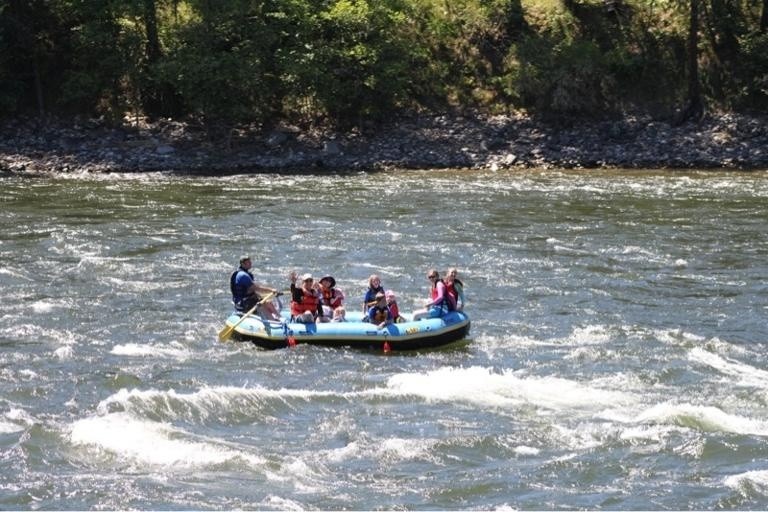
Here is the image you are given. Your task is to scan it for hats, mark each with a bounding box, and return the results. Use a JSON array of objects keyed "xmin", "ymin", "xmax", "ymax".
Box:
[
  {"xmin": 320, "ymin": 275, "xmax": 335, "ymax": 288},
  {"xmin": 303, "ymin": 274, "xmax": 313, "ymax": 281}
]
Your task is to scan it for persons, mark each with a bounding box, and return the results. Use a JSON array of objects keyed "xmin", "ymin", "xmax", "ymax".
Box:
[{"xmin": 230, "ymin": 254, "xmax": 467, "ymax": 330}]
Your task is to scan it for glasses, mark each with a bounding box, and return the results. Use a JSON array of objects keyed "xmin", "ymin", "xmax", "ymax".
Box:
[{"xmin": 429, "ymin": 276, "xmax": 435, "ymax": 278}]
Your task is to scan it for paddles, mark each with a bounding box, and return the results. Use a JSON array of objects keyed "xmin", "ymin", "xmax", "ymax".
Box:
[{"xmin": 219, "ymin": 291, "xmax": 272, "ymax": 342}]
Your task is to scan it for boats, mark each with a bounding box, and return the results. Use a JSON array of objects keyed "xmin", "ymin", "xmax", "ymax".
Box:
[{"xmin": 225, "ymin": 306, "xmax": 474, "ymax": 352}]
